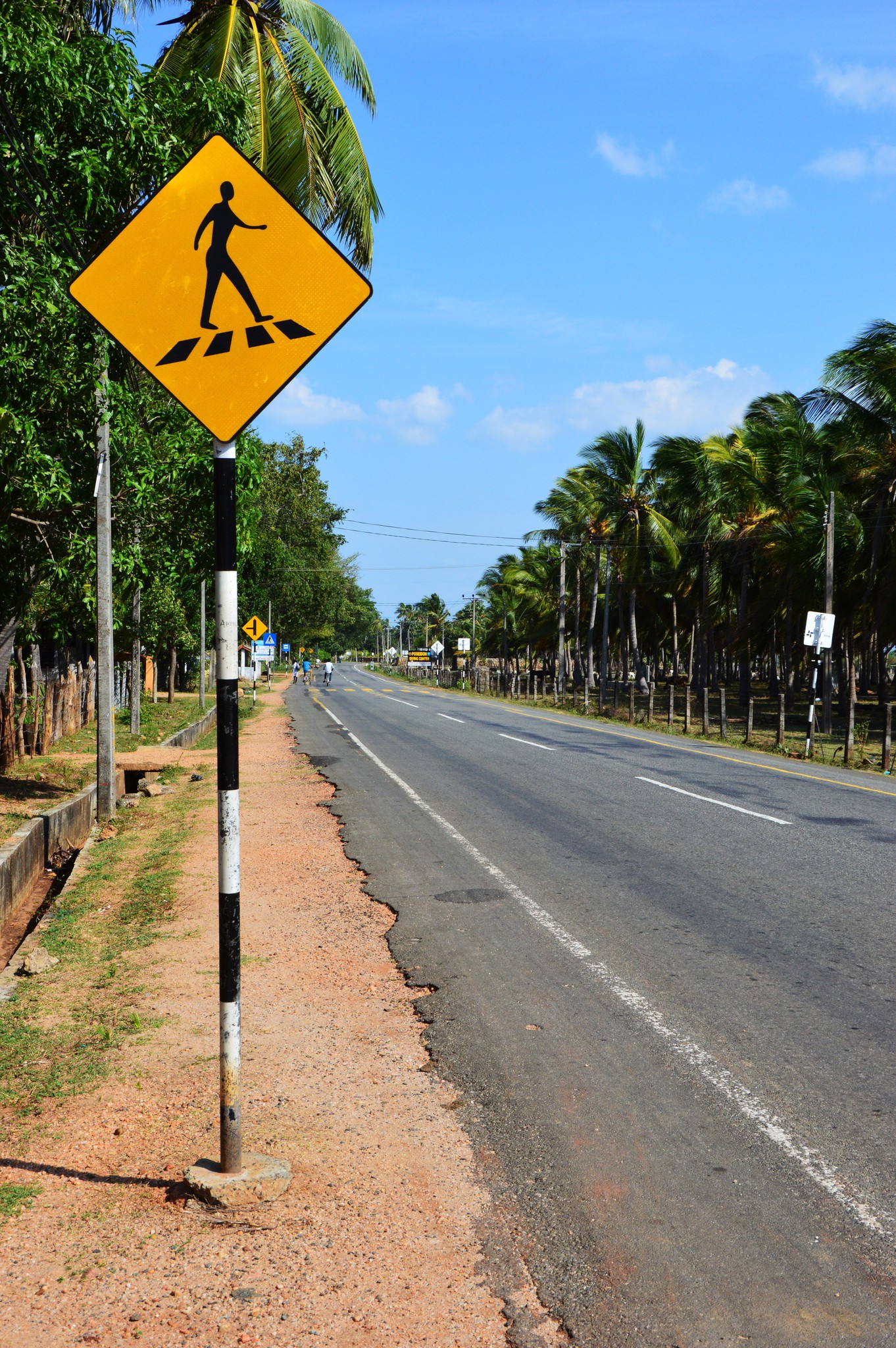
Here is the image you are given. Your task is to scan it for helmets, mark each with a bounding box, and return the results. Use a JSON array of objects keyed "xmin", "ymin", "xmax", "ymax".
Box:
[{"xmin": 317, "ymin": 657, "xmax": 319, "ymax": 659}]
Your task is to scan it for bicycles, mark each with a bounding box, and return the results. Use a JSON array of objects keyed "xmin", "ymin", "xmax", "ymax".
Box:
[
  {"xmin": 303, "ymin": 667, "xmax": 313, "ymax": 685},
  {"xmin": 324, "ymin": 669, "xmax": 332, "ymax": 687}
]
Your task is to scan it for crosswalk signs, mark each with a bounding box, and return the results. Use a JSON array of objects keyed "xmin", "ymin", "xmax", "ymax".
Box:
[{"xmin": 263, "ymin": 633, "xmax": 276, "ymax": 646}]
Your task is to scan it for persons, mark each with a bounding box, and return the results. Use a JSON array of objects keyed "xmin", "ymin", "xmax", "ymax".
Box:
[
  {"xmin": 314, "ymin": 657, "xmax": 321, "ymax": 669},
  {"xmin": 302, "ymin": 658, "xmax": 313, "ymax": 683},
  {"xmin": 323, "ymin": 658, "xmax": 334, "ymax": 683},
  {"xmin": 292, "ymin": 660, "xmax": 300, "ymax": 684}
]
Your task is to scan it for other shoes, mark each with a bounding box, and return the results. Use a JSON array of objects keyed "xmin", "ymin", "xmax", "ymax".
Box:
[
  {"xmin": 329, "ymin": 681, "xmax": 332, "ymax": 682},
  {"xmin": 293, "ymin": 681, "xmax": 295, "ymax": 684},
  {"xmin": 295, "ymin": 683, "xmax": 297, "ymax": 684},
  {"xmin": 314, "ymin": 667, "xmax": 316, "ymax": 668},
  {"xmin": 309, "ymin": 679, "xmax": 313, "ymax": 681},
  {"xmin": 323, "ymin": 680, "xmax": 326, "ymax": 683}
]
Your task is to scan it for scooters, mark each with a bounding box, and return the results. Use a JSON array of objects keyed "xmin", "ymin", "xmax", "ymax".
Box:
[{"xmin": 315, "ymin": 663, "xmax": 319, "ymax": 670}]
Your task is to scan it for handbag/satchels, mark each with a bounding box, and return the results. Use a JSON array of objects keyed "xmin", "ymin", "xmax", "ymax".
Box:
[{"xmin": 296, "ymin": 663, "xmax": 300, "ymax": 671}]
